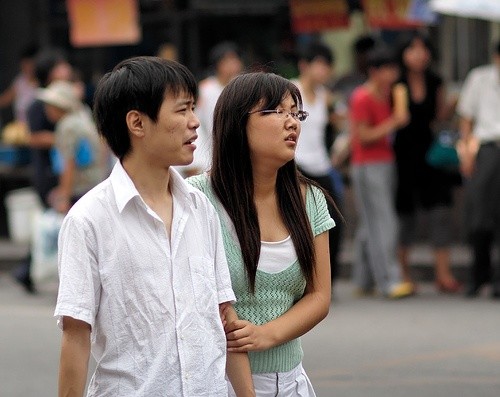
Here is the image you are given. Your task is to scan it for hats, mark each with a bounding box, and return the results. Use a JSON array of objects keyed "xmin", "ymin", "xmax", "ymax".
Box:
[{"xmin": 35, "ymin": 82, "xmax": 74, "ymax": 110}]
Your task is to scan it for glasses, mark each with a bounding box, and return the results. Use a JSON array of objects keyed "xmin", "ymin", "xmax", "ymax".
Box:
[{"xmin": 248, "ymin": 107, "xmax": 309, "ymax": 121}]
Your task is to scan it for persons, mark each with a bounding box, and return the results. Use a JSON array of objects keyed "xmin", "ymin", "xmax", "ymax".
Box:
[
  {"xmin": 0, "ymin": 41, "xmax": 242, "ymax": 292},
  {"xmin": 187, "ymin": 71, "xmax": 336, "ymax": 397},
  {"xmin": 54, "ymin": 55, "xmax": 256, "ymax": 397},
  {"xmin": 292, "ymin": 30, "xmax": 500, "ymax": 302}
]
